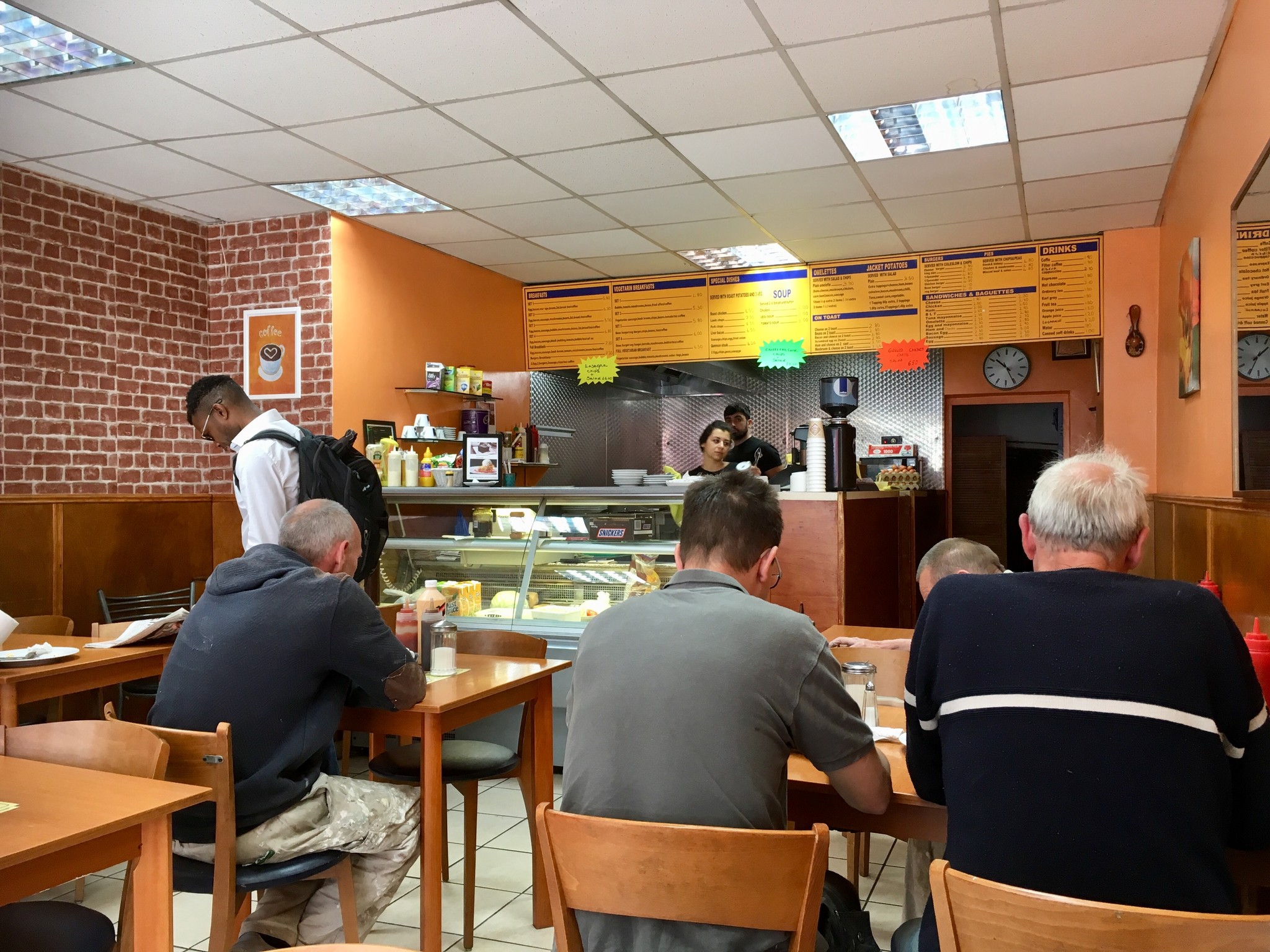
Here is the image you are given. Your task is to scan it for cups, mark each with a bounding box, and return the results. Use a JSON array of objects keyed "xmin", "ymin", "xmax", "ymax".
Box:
[
  {"xmin": 806, "ymin": 418, "xmax": 826, "ymax": 492},
  {"xmin": 457, "ymin": 431, "xmax": 466, "ymax": 440},
  {"xmin": 401, "ymin": 414, "xmax": 436, "ymax": 439},
  {"xmin": 503, "ymin": 473, "xmax": 515, "ymax": 487},
  {"xmin": 736, "ymin": 461, "xmax": 753, "ymax": 476}
]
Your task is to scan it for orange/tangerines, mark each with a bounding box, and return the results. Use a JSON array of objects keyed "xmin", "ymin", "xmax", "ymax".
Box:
[{"xmin": 445, "ymin": 595, "xmax": 482, "ymax": 616}]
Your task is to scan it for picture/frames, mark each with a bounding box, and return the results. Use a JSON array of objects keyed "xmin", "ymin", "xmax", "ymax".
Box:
[
  {"xmin": 363, "ymin": 419, "xmax": 398, "ymax": 451},
  {"xmin": 1052, "ymin": 339, "xmax": 1091, "ymax": 361}
]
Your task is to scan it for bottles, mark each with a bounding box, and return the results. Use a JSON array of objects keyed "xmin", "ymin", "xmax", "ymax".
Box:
[
  {"xmin": 416, "ymin": 580, "xmax": 446, "ymax": 665},
  {"xmin": 429, "ymin": 620, "xmax": 457, "ymax": 677},
  {"xmin": 1243, "ymin": 617, "xmax": 1270, "ymax": 711},
  {"xmin": 388, "ymin": 445, "xmax": 435, "ymax": 487},
  {"xmin": 1197, "ymin": 571, "xmax": 1220, "ymax": 601},
  {"xmin": 395, "ymin": 597, "xmax": 418, "ymax": 653},
  {"xmin": 841, "ymin": 662, "xmax": 877, "ymax": 716},
  {"xmin": 861, "ymin": 681, "xmax": 879, "ymax": 726},
  {"xmin": 511, "ymin": 422, "xmax": 550, "ymax": 463},
  {"xmin": 421, "ymin": 597, "xmax": 443, "ymax": 671}
]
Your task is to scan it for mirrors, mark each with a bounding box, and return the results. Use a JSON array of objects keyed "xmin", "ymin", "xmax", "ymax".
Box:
[{"xmin": 1230, "ymin": 137, "xmax": 1270, "ymax": 498}]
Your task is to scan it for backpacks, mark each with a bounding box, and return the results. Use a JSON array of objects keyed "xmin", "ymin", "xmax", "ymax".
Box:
[{"xmin": 231, "ymin": 425, "xmax": 388, "ymax": 584}]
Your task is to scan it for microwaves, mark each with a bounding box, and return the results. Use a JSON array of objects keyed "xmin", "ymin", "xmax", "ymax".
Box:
[{"xmin": 860, "ymin": 456, "xmax": 924, "ymax": 488}]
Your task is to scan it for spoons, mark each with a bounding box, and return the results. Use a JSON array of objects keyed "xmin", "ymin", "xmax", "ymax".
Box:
[{"xmin": 0, "ymin": 652, "xmax": 36, "ymax": 659}]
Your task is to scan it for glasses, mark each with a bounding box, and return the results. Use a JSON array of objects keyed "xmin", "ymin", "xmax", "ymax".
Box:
[
  {"xmin": 200, "ymin": 399, "xmax": 222, "ymax": 441},
  {"xmin": 760, "ymin": 548, "xmax": 782, "ymax": 589}
]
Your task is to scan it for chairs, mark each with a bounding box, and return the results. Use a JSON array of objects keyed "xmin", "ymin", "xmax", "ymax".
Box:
[{"xmin": 0, "ymin": 579, "xmax": 1270, "ymax": 952}]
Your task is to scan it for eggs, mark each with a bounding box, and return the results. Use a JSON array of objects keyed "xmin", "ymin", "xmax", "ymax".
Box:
[{"xmin": 881, "ymin": 464, "xmax": 917, "ymax": 485}]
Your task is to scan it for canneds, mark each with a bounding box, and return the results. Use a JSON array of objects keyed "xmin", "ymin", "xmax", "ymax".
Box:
[
  {"xmin": 515, "ymin": 447, "xmax": 523, "ymax": 458},
  {"xmin": 539, "ymin": 521, "xmax": 552, "ymax": 539},
  {"xmin": 472, "ymin": 506, "xmax": 493, "ymax": 538},
  {"xmin": 510, "ymin": 512, "xmax": 526, "ymax": 539}
]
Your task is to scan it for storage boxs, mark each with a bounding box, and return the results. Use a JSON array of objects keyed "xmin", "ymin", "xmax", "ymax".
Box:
[{"xmin": 589, "ymin": 516, "xmax": 654, "ymax": 542}]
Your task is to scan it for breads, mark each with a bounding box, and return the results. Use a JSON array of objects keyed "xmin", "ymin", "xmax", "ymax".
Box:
[
  {"xmin": 490, "ymin": 591, "xmax": 530, "ymax": 609},
  {"xmin": 527, "ymin": 592, "xmax": 539, "ymax": 608}
]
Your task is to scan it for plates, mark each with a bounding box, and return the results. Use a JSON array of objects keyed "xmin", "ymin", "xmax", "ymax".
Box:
[
  {"xmin": 435, "ymin": 427, "xmax": 457, "ymax": 440},
  {"xmin": 611, "ymin": 469, "xmax": 676, "ymax": 486},
  {"xmin": 463, "ymin": 482, "xmax": 496, "ymax": 487},
  {"xmin": 0, "ymin": 647, "xmax": 80, "ymax": 667}
]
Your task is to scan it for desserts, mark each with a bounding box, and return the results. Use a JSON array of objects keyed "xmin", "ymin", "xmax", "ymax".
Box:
[
  {"xmin": 478, "ymin": 444, "xmax": 490, "ymax": 453},
  {"xmin": 474, "ymin": 459, "xmax": 495, "ymax": 473}
]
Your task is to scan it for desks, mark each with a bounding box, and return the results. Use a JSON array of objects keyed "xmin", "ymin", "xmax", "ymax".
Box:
[
  {"xmin": 341, "ymin": 654, "xmax": 572, "ymax": 952},
  {"xmin": 819, "ymin": 624, "xmax": 915, "ymax": 652},
  {"xmin": 785, "ymin": 699, "xmax": 948, "ymax": 846},
  {"xmin": 0, "ymin": 631, "xmax": 176, "ymax": 727},
  {"xmin": 0, "ymin": 754, "xmax": 212, "ymax": 952}
]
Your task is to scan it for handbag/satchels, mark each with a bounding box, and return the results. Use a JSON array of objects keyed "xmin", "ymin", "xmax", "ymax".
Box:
[{"xmin": 818, "ymin": 869, "xmax": 881, "ymax": 951}]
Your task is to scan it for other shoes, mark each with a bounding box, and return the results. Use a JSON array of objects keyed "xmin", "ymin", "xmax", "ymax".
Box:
[{"xmin": 229, "ymin": 931, "xmax": 291, "ymax": 952}]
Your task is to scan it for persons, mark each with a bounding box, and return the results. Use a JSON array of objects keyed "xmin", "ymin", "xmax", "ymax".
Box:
[
  {"xmin": 826, "ymin": 538, "xmax": 1015, "ymax": 651},
  {"xmin": 146, "ymin": 500, "xmax": 430, "ymax": 952},
  {"xmin": 681, "ymin": 420, "xmax": 762, "ymax": 481},
  {"xmin": 554, "ymin": 474, "xmax": 891, "ymax": 952},
  {"xmin": 890, "ymin": 444, "xmax": 1270, "ymax": 952},
  {"xmin": 723, "ymin": 402, "xmax": 784, "ymax": 479},
  {"xmin": 186, "ymin": 376, "xmax": 315, "ymax": 554}
]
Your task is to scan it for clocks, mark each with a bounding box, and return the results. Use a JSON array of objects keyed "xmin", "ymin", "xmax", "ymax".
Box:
[
  {"xmin": 982, "ymin": 345, "xmax": 1031, "ymax": 390},
  {"xmin": 1237, "ymin": 332, "xmax": 1270, "ymax": 382}
]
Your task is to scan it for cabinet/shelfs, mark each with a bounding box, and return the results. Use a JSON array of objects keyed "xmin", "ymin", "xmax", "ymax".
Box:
[{"xmin": 395, "ymin": 387, "xmax": 503, "ymax": 444}]
